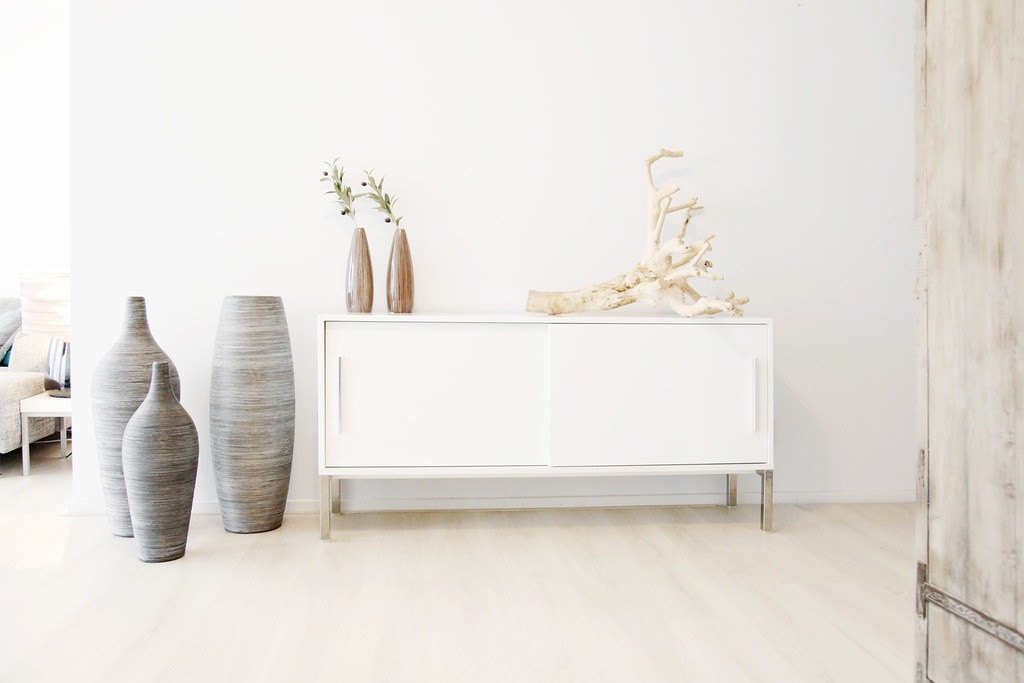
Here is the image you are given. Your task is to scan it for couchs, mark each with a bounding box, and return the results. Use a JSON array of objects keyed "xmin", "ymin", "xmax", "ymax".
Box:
[{"xmin": 0, "ymin": 297, "xmax": 72, "ymax": 453}]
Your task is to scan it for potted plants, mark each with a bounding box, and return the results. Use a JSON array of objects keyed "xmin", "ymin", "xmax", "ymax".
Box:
[
  {"xmin": 355, "ymin": 168, "xmax": 414, "ymax": 315},
  {"xmin": 319, "ymin": 156, "xmax": 375, "ymax": 314}
]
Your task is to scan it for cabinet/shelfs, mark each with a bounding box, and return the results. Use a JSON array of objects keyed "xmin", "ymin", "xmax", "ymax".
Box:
[{"xmin": 315, "ymin": 312, "xmax": 776, "ymax": 541}]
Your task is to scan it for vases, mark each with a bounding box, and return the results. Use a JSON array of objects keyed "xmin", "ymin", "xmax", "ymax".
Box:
[
  {"xmin": 89, "ymin": 297, "xmax": 181, "ymax": 539},
  {"xmin": 120, "ymin": 362, "xmax": 200, "ymax": 562},
  {"xmin": 209, "ymin": 295, "xmax": 296, "ymax": 534}
]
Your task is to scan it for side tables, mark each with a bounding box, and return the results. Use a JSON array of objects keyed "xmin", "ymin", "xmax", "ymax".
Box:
[{"xmin": 20, "ymin": 392, "xmax": 73, "ymax": 476}]
